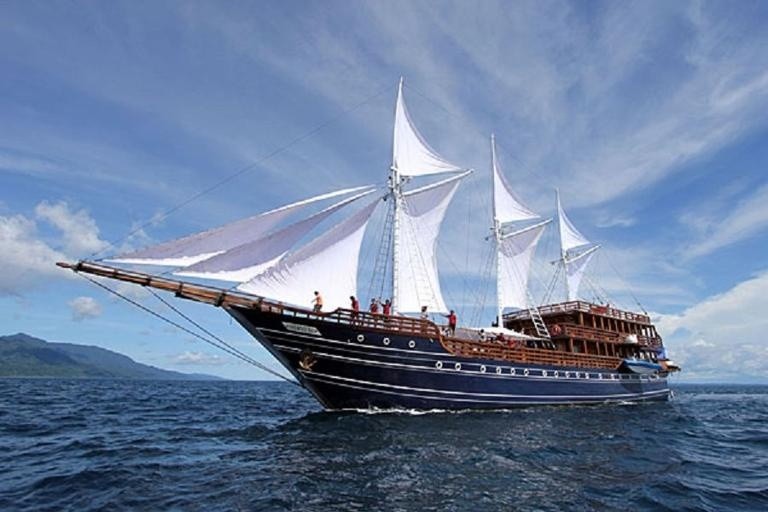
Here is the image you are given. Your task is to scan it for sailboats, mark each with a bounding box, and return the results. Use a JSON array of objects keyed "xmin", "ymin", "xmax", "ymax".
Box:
[{"xmin": 55, "ymin": 74, "xmax": 681, "ymax": 413}]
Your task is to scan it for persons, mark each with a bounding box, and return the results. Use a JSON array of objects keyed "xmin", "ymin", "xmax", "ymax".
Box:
[
  {"xmin": 367, "ymin": 298, "xmax": 379, "ymax": 328},
  {"xmin": 311, "ymin": 291, "xmax": 323, "ymax": 320},
  {"xmin": 379, "ymin": 297, "xmax": 393, "ymax": 330},
  {"xmin": 495, "ymin": 333, "xmax": 506, "ymax": 357},
  {"xmin": 479, "ymin": 328, "xmax": 487, "ymax": 344},
  {"xmin": 440, "ymin": 310, "xmax": 456, "ymax": 337},
  {"xmin": 502, "ymin": 336, "xmax": 515, "ymax": 359},
  {"xmin": 420, "ymin": 306, "xmax": 429, "ymax": 334},
  {"xmin": 349, "ymin": 296, "xmax": 361, "ymax": 325}
]
